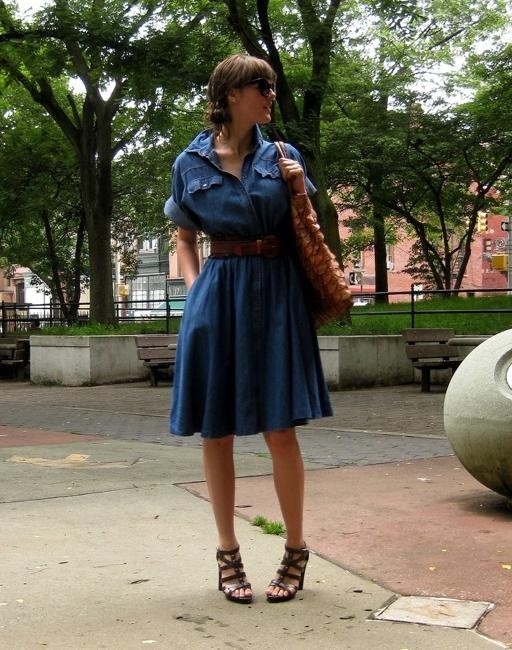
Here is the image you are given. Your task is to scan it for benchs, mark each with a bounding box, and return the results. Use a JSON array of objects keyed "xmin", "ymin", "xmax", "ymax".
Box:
[
  {"xmin": 403, "ymin": 329, "xmax": 463, "ymax": 392},
  {"xmin": 1, "ymin": 338, "xmax": 28, "ymax": 377},
  {"xmin": 135, "ymin": 336, "xmax": 177, "ymax": 386}
]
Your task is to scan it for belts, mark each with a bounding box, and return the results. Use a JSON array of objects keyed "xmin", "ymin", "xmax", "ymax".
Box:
[{"xmin": 200, "ymin": 237, "xmax": 285, "ymax": 258}]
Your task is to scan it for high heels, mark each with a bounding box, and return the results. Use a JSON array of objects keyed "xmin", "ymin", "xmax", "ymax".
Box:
[
  {"xmin": 215, "ymin": 544, "xmax": 253, "ymax": 604},
  {"xmin": 266, "ymin": 544, "xmax": 309, "ymax": 603}
]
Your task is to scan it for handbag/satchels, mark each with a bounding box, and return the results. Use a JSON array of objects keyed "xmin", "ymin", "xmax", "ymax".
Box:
[{"xmin": 273, "ymin": 139, "xmax": 354, "ymax": 328}]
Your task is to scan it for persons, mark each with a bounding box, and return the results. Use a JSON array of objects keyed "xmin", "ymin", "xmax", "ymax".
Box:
[{"xmin": 159, "ymin": 51, "xmax": 353, "ymax": 603}]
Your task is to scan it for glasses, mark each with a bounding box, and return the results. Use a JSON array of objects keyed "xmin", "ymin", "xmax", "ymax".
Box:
[{"xmin": 229, "ymin": 77, "xmax": 277, "ymax": 96}]
